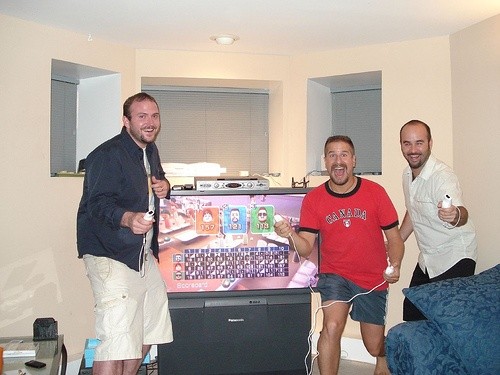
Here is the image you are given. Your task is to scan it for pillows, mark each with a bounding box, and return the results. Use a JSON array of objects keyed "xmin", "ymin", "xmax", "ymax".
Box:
[{"xmin": 402, "ymin": 264, "xmax": 499, "ymax": 374}]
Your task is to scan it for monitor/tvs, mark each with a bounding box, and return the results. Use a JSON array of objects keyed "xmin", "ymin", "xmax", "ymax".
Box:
[{"xmin": 149, "ymin": 189, "xmax": 320, "ymax": 306}]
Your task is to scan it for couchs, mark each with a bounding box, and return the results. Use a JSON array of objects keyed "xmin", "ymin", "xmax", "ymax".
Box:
[{"xmin": 383, "ymin": 320, "xmax": 466, "ymax": 375}]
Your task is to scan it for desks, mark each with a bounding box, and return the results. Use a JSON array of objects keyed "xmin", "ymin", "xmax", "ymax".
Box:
[{"xmin": 156, "ymin": 294, "xmax": 311, "ymax": 374}]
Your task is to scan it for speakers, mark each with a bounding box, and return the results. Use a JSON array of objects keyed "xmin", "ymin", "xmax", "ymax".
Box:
[{"xmin": 157, "ymin": 303, "xmax": 316, "ymax": 375}]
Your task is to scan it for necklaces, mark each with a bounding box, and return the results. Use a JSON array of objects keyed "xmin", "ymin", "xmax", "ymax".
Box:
[{"xmin": 329, "ymin": 177, "xmax": 356, "ymax": 194}]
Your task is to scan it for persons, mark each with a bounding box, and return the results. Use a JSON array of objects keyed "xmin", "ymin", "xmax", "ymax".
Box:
[
  {"xmin": 274, "ymin": 136, "xmax": 406, "ymax": 375},
  {"xmin": 385, "ymin": 120, "xmax": 478, "ymax": 320},
  {"xmin": 76, "ymin": 92, "xmax": 171, "ymax": 374}
]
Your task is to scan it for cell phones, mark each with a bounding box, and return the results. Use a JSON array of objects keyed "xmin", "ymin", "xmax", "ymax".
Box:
[{"xmin": 184, "ymin": 184, "xmax": 193, "ymax": 190}]
